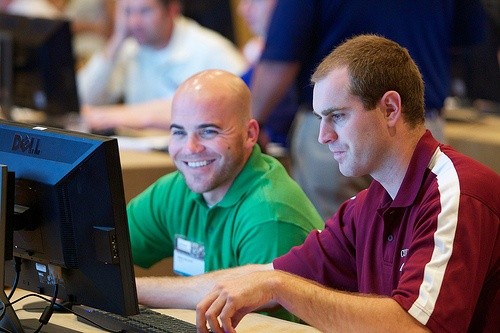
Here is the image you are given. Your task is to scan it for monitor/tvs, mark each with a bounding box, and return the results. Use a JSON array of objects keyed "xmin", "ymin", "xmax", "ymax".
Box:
[
  {"xmin": 0, "ymin": 117, "xmax": 140, "ymax": 333},
  {"xmin": 0, "ymin": 12, "xmax": 80, "ymax": 120}
]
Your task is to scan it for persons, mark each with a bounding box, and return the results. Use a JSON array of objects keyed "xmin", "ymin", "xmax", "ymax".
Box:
[
  {"xmin": 127, "ymin": 69, "xmax": 325, "ymax": 326},
  {"xmin": 251, "ymin": 0, "xmax": 451, "ymax": 223},
  {"xmin": 136, "ymin": 33, "xmax": 500, "ymax": 333},
  {"xmin": 78, "ymin": 0, "xmax": 298, "ymax": 150},
  {"xmin": 76, "ymin": 0, "xmax": 248, "ymax": 106}
]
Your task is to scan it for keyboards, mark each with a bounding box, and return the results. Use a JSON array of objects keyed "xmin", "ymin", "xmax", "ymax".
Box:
[{"xmin": 71, "ymin": 304, "xmax": 213, "ymax": 333}]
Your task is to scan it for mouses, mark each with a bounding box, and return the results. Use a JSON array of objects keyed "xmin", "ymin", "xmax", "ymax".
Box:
[{"xmin": 53, "ymin": 301, "xmax": 80, "ymax": 312}]
